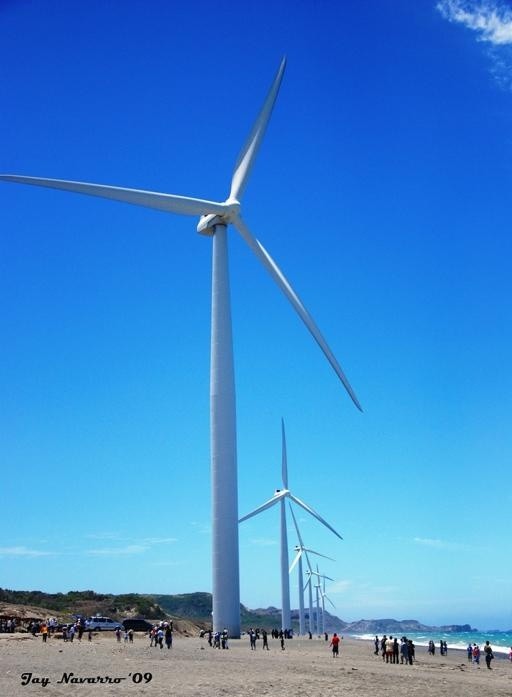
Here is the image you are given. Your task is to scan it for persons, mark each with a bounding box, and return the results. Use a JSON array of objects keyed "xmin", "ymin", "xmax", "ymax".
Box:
[
  {"xmin": 199, "ymin": 627, "xmax": 343, "ymax": 658},
  {"xmin": 373, "ymin": 635, "xmax": 512, "ymax": 670},
  {"xmin": 0, "ymin": 614, "xmax": 174, "ymax": 649}
]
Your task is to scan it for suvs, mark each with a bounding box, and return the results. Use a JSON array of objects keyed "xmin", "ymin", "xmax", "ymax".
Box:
[
  {"xmin": 122, "ymin": 619, "xmax": 155, "ymax": 631},
  {"xmin": 85, "ymin": 615, "xmax": 125, "ymax": 631}
]
